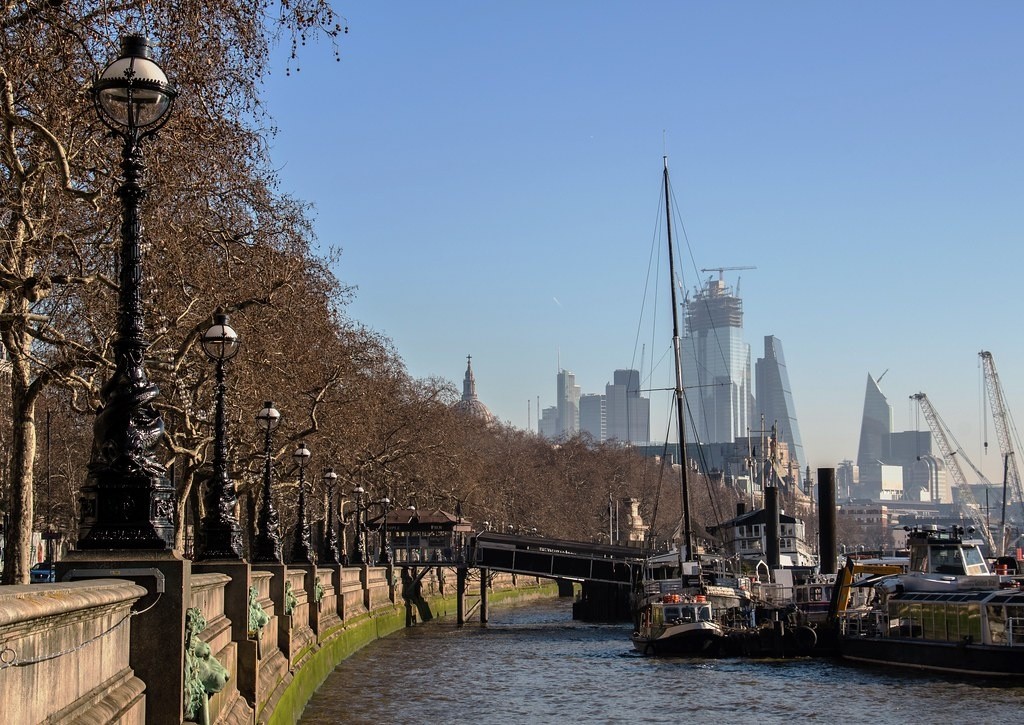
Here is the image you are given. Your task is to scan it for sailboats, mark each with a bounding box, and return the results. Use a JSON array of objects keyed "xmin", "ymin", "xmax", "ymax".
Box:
[{"xmin": 630, "ymin": 129, "xmax": 876, "ymax": 660}]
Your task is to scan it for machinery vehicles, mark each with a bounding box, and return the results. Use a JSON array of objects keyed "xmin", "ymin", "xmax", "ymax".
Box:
[{"xmin": 906, "ymin": 390, "xmax": 997, "ymax": 554}]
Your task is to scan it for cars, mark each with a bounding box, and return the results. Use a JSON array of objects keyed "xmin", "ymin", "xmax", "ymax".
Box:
[{"xmin": 29, "ymin": 562, "xmax": 54, "ymax": 583}]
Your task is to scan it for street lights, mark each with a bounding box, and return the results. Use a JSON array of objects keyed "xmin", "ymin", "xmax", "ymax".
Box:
[
  {"xmin": 292, "ymin": 441, "xmax": 314, "ymax": 563},
  {"xmin": 353, "ymin": 483, "xmax": 366, "ymax": 563},
  {"xmin": 324, "ymin": 467, "xmax": 341, "ymax": 565},
  {"xmin": 379, "ymin": 494, "xmax": 392, "ymax": 564},
  {"xmin": 197, "ymin": 305, "xmax": 245, "ymax": 562},
  {"xmin": 80, "ymin": 30, "xmax": 180, "ymax": 548},
  {"xmin": 258, "ymin": 399, "xmax": 283, "ymax": 564}
]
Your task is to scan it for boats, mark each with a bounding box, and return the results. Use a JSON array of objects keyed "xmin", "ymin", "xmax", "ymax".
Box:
[{"xmin": 839, "ymin": 451, "xmax": 1024, "ymax": 686}]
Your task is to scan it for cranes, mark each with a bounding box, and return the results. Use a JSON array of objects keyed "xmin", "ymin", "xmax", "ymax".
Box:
[
  {"xmin": 700, "ymin": 266, "xmax": 757, "ymax": 280},
  {"xmin": 974, "ymin": 349, "xmax": 1023, "ymax": 512}
]
